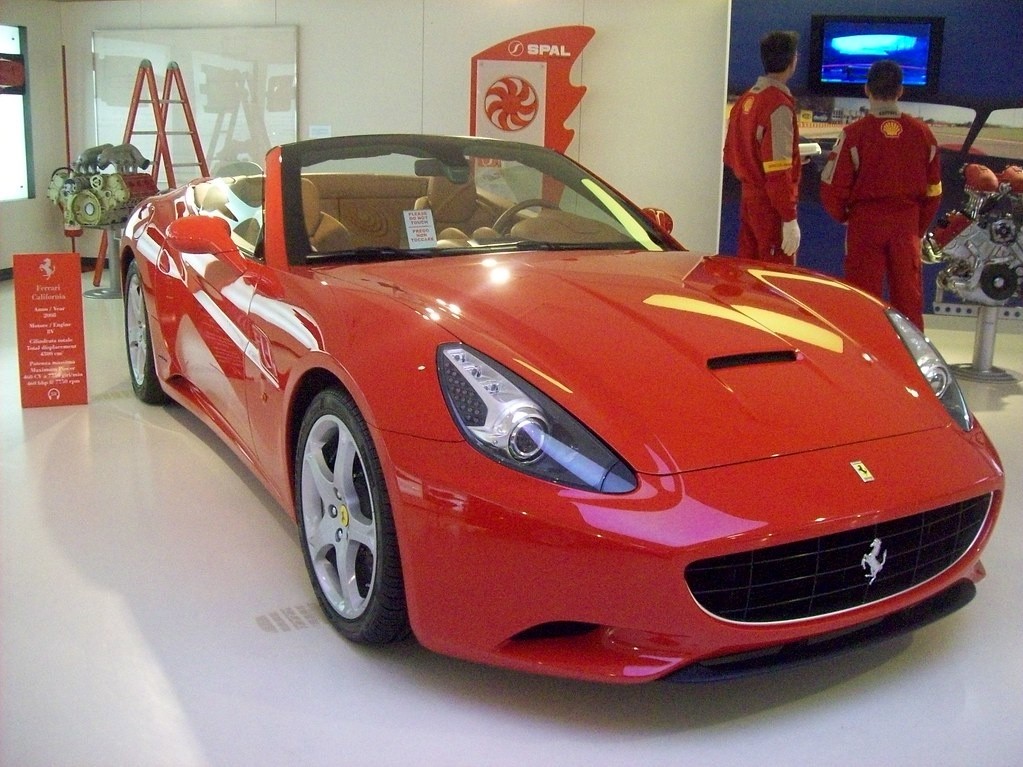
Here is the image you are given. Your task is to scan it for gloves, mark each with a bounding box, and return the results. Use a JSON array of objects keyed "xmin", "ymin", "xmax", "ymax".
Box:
[
  {"xmin": 800, "ymin": 156, "xmax": 811, "ymax": 165},
  {"xmin": 781, "ymin": 219, "xmax": 801, "ymax": 256}
]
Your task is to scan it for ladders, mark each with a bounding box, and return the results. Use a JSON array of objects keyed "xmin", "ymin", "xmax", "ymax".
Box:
[{"xmin": 92, "ymin": 58, "xmax": 210, "ymax": 286}]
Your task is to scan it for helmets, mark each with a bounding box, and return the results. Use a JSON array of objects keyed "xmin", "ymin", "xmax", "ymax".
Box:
[
  {"xmin": 963, "ymin": 163, "xmax": 998, "ymax": 192},
  {"xmin": 1000, "ymin": 165, "xmax": 1023, "ymax": 193}
]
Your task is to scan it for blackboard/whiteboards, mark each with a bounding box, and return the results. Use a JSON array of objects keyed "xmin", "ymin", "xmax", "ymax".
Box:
[{"xmin": 90, "ymin": 24, "xmax": 300, "ymax": 192}]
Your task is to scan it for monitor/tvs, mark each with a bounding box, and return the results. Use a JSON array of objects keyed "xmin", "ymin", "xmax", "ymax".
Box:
[{"xmin": 807, "ymin": 12, "xmax": 946, "ymax": 102}]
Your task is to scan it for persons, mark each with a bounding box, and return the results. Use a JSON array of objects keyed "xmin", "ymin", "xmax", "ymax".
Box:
[
  {"xmin": 820, "ymin": 60, "xmax": 942, "ymax": 333},
  {"xmin": 723, "ymin": 33, "xmax": 810, "ymax": 267}
]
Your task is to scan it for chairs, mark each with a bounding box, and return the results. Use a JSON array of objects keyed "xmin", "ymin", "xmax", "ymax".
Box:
[
  {"xmin": 248, "ymin": 179, "xmax": 353, "ymax": 256},
  {"xmin": 415, "ymin": 163, "xmax": 497, "ymax": 248}
]
